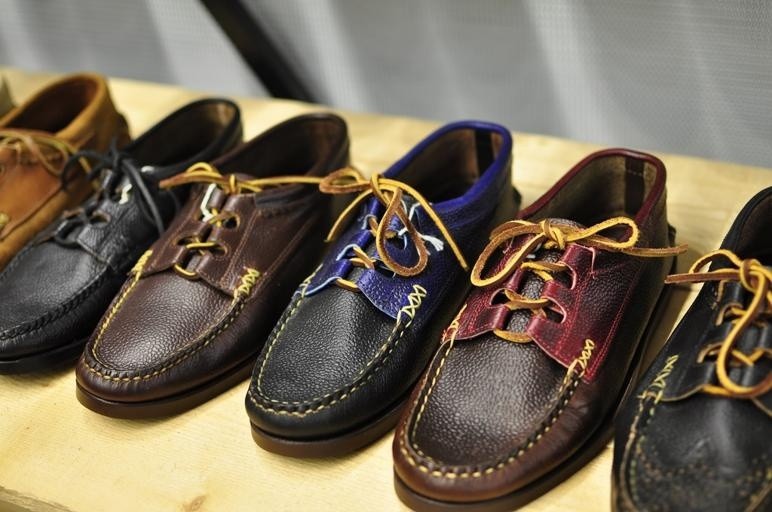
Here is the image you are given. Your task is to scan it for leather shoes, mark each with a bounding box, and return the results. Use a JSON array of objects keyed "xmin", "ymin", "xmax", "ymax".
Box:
[
  {"xmin": 610, "ymin": 187, "xmax": 771, "ymax": 511},
  {"xmin": 3, "ymin": 74, "xmax": 132, "ymax": 270},
  {"xmin": 245, "ymin": 119, "xmax": 520, "ymax": 460},
  {"xmin": 3, "ymin": 98, "xmax": 243, "ymax": 376},
  {"xmin": 391, "ymin": 146, "xmax": 689, "ymax": 508},
  {"xmin": 74, "ymin": 112, "xmax": 364, "ymax": 418}
]
